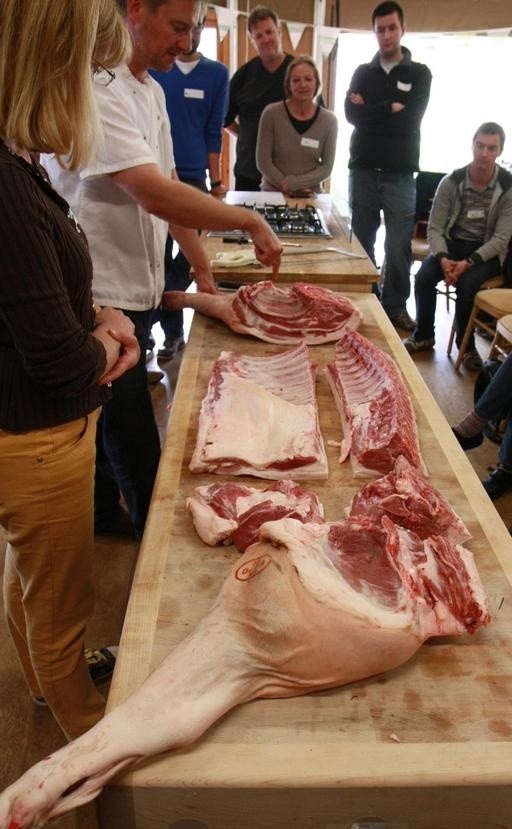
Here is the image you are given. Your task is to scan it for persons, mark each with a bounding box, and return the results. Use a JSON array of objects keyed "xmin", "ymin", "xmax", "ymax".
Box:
[
  {"xmin": 223, "ymin": 4, "xmax": 325, "ymax": 194},
  {"xmin": 253, "ymin": 54, "xmax": 337, "ymax": 195},
  {"xmin": 452, "ymin": 350, "xmax": 512, "ymax": 502},
  {"xmin": 36, "ymin": 0, "xmax": 283, "ymax": 545},
  {"xmin": 134, "ymin": 0, "xmax": 233, "ymax": 360},
  {"xmin": 0, "ymin": 1, "xmax": 142, "ymax": 755},
  {"xmin": 395, "ymin": 120, "xmax": 512, "ymax": 375},
  {"xmin": 345, "ymin": 0, "xmax": 431, "ymax": 332}
]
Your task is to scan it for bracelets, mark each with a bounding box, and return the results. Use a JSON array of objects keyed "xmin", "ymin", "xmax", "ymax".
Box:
[{"xmin": 209, "ymin": 180, "xmax": 223, "ymax": 189}]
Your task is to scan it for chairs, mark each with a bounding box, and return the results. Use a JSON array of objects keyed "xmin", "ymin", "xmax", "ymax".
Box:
[{"xmin": 410, "ymin": 168, "xmax": 512, "ymax": 431}]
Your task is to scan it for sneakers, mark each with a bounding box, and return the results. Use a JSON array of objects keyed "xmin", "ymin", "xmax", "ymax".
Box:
[
  {"xmin": 399, "ymin": 329, "xmax": 435, "ymax": 352},
  {"xmin": 33, "ymin": 645, "xmax": 119, "ymax": 706},
  {"xmin": 461, "ymin": 343, "xmax": 483, "ymax": 370},
  {"xmin": 391, "ymin": 306, "xmax": 417, "ymax": 330},
  {"xmin": 146, "ymin": 334, "xmax": 186, "ymax": 384},
  {"xmin": 482, "ymin": 468, "xmax": 512, "ymax": 501},
  {"xmin": 451, "ymin": 425, "xmax": 483, "ymax": 450}
]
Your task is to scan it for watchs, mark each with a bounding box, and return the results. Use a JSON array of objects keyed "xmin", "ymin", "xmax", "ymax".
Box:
[{"xmin": 465, "ymin": 258, "xmax": 474, "ymax": 268}]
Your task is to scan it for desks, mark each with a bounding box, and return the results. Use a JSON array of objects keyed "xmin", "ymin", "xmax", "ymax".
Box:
[
  {"xmin": 96, "ymin": 294, "xmax": 512, "ymax": 829},
  {"xmin": 188, "ymin": 193, "xmax": 379, "ymax": 292}
]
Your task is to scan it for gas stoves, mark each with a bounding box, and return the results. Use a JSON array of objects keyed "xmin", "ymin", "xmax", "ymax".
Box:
[{"xmin": 202, "ymin": 200, "xmax": 331, "ymax": 241}]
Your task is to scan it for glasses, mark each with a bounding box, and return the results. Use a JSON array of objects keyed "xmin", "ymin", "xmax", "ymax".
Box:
[{"xmin": 86, "ymin": 51, "xmax": 116, "ymax": 87}]
